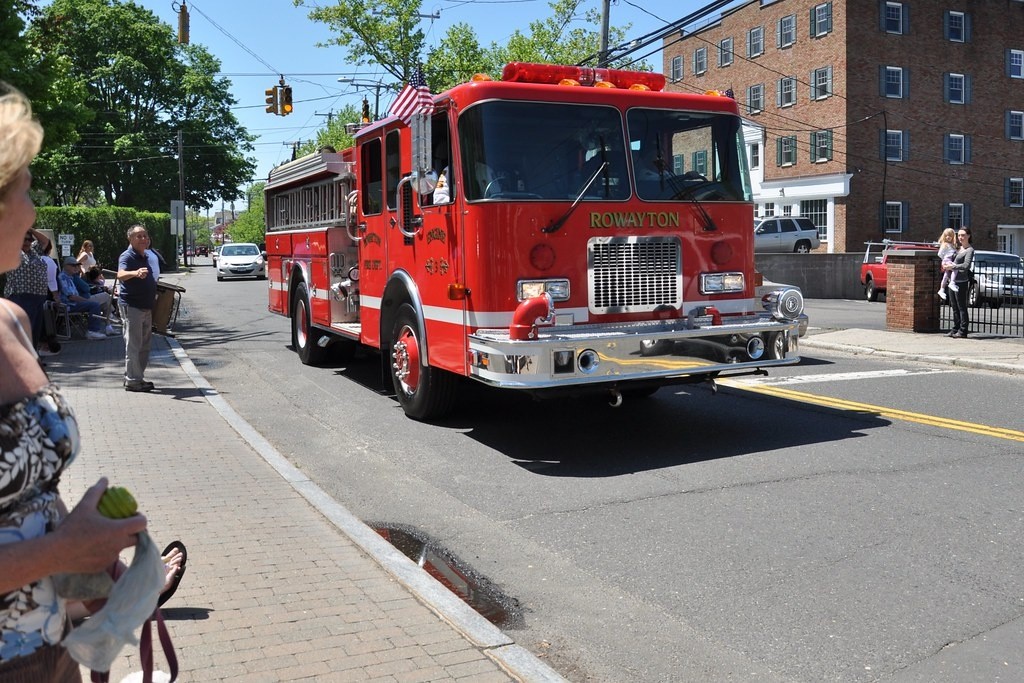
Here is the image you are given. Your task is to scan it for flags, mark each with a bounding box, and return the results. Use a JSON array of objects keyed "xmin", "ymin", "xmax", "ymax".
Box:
[{"xmin": 388, "ymin": 66, "xmax": 434, "ymax": 127}]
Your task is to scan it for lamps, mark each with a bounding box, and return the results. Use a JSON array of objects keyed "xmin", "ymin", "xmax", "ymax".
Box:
[{"xmin": 780, "ymin": 188, "xmax": 784, "ymax": 196}]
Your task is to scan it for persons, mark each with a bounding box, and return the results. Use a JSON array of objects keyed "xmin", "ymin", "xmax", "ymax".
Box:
[
  {"xmin": 0, "ymin": 91, "xmax": 148, "ymax": 683},
  {"xmin": 3, "ymin": 228, "xmax": 61, "ymax": 356},
  {"xmin": 56, "ymin": 239, "xmax": 123, "ymax": 338},
  {"xmin": 432, "ymin": 140, "xmax": 502, "ymax": 204},
  {"xmin": 64, "ymin": 547, "xmax": 184, "ymax": 621},
  {"xmin": 936, "ymin": 226, "xmax": 974, "ymax": 338},
  {"xmin": 114, "ymin": 222, "xmax": 167, "ymax": 391},
  {"xmin": 638, "ymin": 144, "xmax": 699, "ymax": 185}
]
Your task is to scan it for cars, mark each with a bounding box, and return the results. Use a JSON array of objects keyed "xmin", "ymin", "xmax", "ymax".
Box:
[
  {"xmin": 211, "ymin": 245, "xmax": 223, "ymax": 268},
  {"xmin": 966, "ymin": 249, "xmax": 1024, "ymax": 308},
  {"xmin": 213, "ymin": 241, "xmax": 267, "ymax": 281},
  {"xmin": 178, "ymin": 243, "xmax": 209, "ymax": 257}
]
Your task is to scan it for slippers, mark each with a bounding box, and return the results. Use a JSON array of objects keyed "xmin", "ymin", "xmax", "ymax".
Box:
[{"xmin": 156, "ymin": 540, "xmax": 187, "ymax": 608}]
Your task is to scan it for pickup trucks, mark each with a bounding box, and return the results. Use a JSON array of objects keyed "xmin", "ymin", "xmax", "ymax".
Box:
[{"xmin": 860, "ymin": 239, "xmax": 951, "ymax": 305}]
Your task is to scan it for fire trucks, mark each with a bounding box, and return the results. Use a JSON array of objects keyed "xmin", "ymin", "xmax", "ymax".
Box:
[{"xmin": 262, "ymin": 59, "xmax": 808, "ymax": 424}]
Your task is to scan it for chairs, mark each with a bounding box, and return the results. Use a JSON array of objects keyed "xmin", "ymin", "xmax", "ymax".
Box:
[
  {"xmin": 44, "ymin": 300, "xmax": 88, "ymax": 339},
  {"xmin": 92, "ymin": 268, "xmax": 186, "ymax": 339}
]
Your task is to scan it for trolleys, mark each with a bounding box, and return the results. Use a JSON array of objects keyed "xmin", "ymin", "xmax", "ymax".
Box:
[{"xmin": 88, "ymin": 267, "xmax": 186, "ymax": 339}]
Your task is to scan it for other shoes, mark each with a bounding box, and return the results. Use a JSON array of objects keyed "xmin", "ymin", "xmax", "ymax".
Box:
[
  {"xmin": 948, "ymin": 283, "xmax": 958, "ymax": 292},
  {"xmin": 943, "ymin": 331, "xmax": 955, "ymax": 336},
  {"xmin": 38, "ymin": 349, "xmax": 57, "ymax": 356},
  {"xmin": 938, "ymin": 291, "xmax": 947, "ymax": 299},
  {"xmin": 952, "ymin": 332, "xmax": 967, "ymax": 338},
  {"xmin": 86, "ymin": 330, "xmax": 107, "ymax": 339},
  {"xmin": 106, "ymin": 329, "xmax": 121, "ymax": 335},
  {"xmin": 124, "ymin": 381, "xmax": 155, "ymax": 391}
]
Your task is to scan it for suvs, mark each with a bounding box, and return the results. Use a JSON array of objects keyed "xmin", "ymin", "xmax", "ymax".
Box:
[{"xmin": 753, "ymin": 214, "xmax": 820, "ymax": 254}]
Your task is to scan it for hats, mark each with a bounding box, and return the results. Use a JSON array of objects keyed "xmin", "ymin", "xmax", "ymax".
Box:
[{"xmin": 64, "ymin": 257, "xmax": 82, "ymax": 265}]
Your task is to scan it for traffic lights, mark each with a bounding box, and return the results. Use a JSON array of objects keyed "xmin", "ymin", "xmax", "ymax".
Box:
[
  {"xmin": 362, "ymin": 102, "xmax": 369, "ymax": 122},
  {"xmin": 280, "ymin": 86, "xmax": 293, "ymax": 114},
  {"xmin": 265, "ymin": 86, "xmax": 278, "ymax": 116}
]
